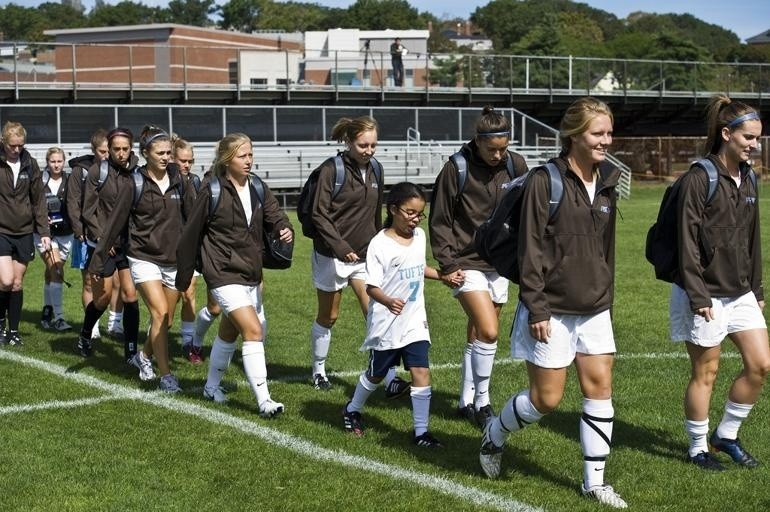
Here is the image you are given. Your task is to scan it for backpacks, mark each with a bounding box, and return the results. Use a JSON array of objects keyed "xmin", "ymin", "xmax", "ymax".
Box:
[
  {"xmin": 195, "ymin": 172, "xmax": 266, "ymax": 274},
  {"xmin": 647, "ymin": 159, "xmax": 760, "ymax": 283},
  {"xmin": 474, "ymin": 163, "xmax": 564, "ymax": 286},
  {"xmin": 296, "ymin": 153, "xmax": 383, "ymax": 238}
]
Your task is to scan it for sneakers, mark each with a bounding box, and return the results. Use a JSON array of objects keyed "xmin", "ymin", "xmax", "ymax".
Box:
[
  {"xmin": 41, "ymin": 320, "xmax": 52, "ymax": 328},
  {"xmin": 259, "ymin": 399, "xmax": 283, "ymax": 418},
  {"xmin": 0, "ymin": 329, "xmax": 9, "ymax": 346},
  {"xmin": 160, "ymin": 375, "xmax": 182, "ymax": 393},
  {"xmin": 78, "ymin": 332, "xmax": 90, "ymax": 357},
  {"xmin": 106, "ymin": 323, "xmax": 124, "ymax": 337},
  {"xmin": 312, "ymin": 375, "xmax": 334, "ymax": 393},
  {"xmin": 458, "ymin": 404, "xmax": 479, "ymax": 426},
  {"xmin": 581, "ymin": 483, "xmax": 627, "ymax": 510},
  {"xmin": 9, "ymin": 332, "xmax": 23, "ymax": 346},
  {"xmin": 133, "ymin": 351, "xmax": 156, "ymax": 382},
  {"xmin": 686, "ymin": 450, "xmax": 727, "ymax": 472},
  {"xmin": 480, "ymin": 417, "xmax": 504, "ymax": 480},
  {"xmin": 54, "ymin": 318, "xmax": 70, "ymax": 330},
  {"xmin": 189, "ymin": 340, "xmax": 202, "ymax": 365},
  {"xmin": 474, "ymin": 403, "xmax": 497, "ymax": 428},
  {"xmin": 343, "ymin": 401, "xmax": 363, "ymax": 438},
  {"xmin": 91, "ymin": 329, "xmax": 100, "ymax": 341},
  {"xmin": 710, "ymin": 430, "xmax": 760, "ymax": 468},
  {"xmin": 125, "ymin": 353, "xmax": 137, "ymax": 366},
  {"xmin": 203, "ymin": 385, "xmax": 227, "ymax": 405},
  {"xmin": 385, "ymin": 379, "xmax": 413, "ymax": 399},
  {"xmin": 413, "ymin": 432, "xmax": 442, "ymax": 450}
]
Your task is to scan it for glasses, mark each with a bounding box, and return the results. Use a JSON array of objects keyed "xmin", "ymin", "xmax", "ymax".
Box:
[{"xmin": 395, "ymin": 205, "xmax": 427, "ymax": 221}]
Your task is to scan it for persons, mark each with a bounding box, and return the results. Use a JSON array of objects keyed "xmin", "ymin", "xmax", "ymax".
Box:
[
  {"xmin": 148, "ymin": 136, "xmax": 202, "ymax": 355},
  {"xmin": 645, "ymin": 94, "xmax": 770, "ymax": 472},
  {"xmin": 0, "ymin": 122, "xmax": 52, "ymax": 347},
  {"xmin": 297, "ymin": 115, "xmax": 413, "ymax": 401},
  {"xmin": 35, "ymin": 148, "xmax": 75, "ymax": 330},
  {"xmin": 175, "ymin": 132, "xmax": 294, "ymax": 417},
  {"xmin": 88, "ymin": 125, "xmax": 196, "ymax": 393},
  {"xmin": 390, "ymin": 37, "xmax": 407, "ymax": 87},
  {"xmin": 77, "ymin": 129, "xmax": 141, "ymax": 367},
  {"xmin": 429, "ymin": 106, "xmax": 529, "ymax": 425},
  {"xmin": 67, "ymin": 129, "xmax": 124, "ymax": 345},
  {"xmin": 479, "ymin": 98, "xmax": 629, "ymax": 508},
  {"xmin": 341, "ymin": 182, "xmax": 465, "ymax": 448}
]
